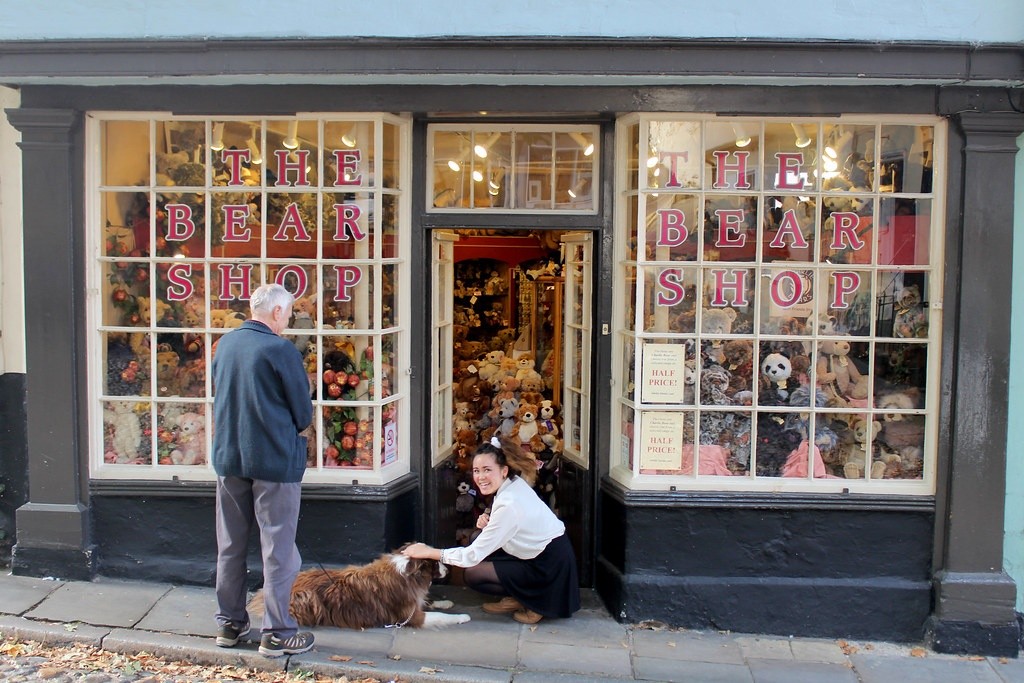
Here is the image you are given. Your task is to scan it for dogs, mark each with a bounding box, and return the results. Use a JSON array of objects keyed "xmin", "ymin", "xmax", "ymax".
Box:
[{"xmin": 245, "ymin": 540, "xmax": 471, "ymax": 627}]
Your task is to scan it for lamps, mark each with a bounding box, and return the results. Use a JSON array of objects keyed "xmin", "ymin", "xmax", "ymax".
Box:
[
  {"xmin": 826, "ymin": 215, "xmax": 887, "ymax": 265},
  {"xmin": 208, "ymin": 121, "xmax": 854, "ymax": 202}
]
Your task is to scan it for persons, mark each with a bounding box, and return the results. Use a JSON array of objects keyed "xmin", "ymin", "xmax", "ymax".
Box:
[
  {"xmin": 210, "ymin": 284, "xmax": 315, "ymax": 657},
  {"xmin": 401, "ymin": 442, "xmax": 582, "ymax": 625}
]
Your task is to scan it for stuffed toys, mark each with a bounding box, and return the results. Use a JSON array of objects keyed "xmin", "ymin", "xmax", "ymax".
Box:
[
  {"xmin": 620, "ymin": 163, "xmax": 928, "ymax": 480},
  {"xmin": 104, "ymin": 293, "xmax": 389, "ymax": 465},
  {"xmin": 449, "ymin": 256, "xmax": 563, "ymax": 545}
]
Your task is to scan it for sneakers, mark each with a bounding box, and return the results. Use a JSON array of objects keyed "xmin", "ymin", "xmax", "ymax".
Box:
[
  {"xmin": 213, "ymin": 620, "xmax": 252, "ymax": 648},
  {"xmin": 481, "ymin": 593, "xmax": 545, "ymax": 624},
  {"xmin": 258, "ymin": 628, "xmax": 316, "ymax": 659}
]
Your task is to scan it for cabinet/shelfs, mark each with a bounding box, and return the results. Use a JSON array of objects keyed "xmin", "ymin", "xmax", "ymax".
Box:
[{"xmin": 161, "ymin": 224, "xmax": 516, "ymax": 332}]
